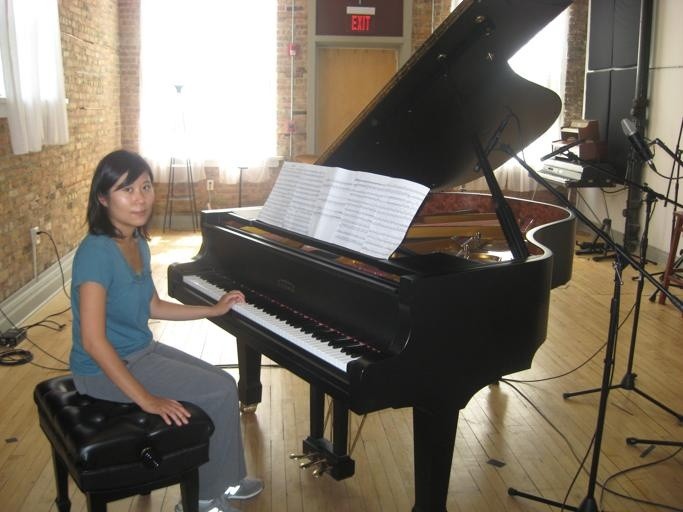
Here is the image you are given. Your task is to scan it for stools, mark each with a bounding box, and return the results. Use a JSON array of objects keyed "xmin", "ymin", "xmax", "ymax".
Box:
[{"xmin": 33, "ymin": 371, "xmax": 216, "ymax": 512}]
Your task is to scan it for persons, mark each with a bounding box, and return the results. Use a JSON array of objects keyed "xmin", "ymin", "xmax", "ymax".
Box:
[{"xmin": 69, "ymin": 150, "xmax": 266, "ymax": 512}]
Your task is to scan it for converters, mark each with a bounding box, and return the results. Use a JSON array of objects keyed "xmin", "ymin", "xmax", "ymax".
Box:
[{"xmin": 0, "ymin": 328, "xmax": 27, "ymax": 346}]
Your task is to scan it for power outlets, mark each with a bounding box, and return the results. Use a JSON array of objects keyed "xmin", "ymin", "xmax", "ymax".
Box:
[
  {"xmin": 30, "ymin": 226, "xmax": 41, "ymax": 245},
  {"xmin": 206, "ymin": 179, "xmax": 214, "ymax": 190}
]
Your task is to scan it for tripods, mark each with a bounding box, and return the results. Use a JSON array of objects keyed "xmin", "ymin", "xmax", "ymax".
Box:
[{"xmin": 501, "ymin": 138, "xmax": 683, "ymax": 512}]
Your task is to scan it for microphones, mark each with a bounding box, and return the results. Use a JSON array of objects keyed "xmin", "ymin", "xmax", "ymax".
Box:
[
  {"xmin": 621, "ymin": 118, "xmax": 654, "ymax": 162},
  {"xmin": 473, "ymin": 114, "xmax": 507, "ymax": 173},
  {"xmin": 540, "ymin": 137, "xmax": 588, "ymax": 163}
]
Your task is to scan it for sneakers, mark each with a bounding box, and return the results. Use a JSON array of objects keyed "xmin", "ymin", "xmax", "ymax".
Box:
[
  {"xmin": 224, "ymin": 477, "xmax": 265, "ymax": 499},
  {"xmin": 173, "ymin": 495, "xmax": 246, "ymax": 512}
]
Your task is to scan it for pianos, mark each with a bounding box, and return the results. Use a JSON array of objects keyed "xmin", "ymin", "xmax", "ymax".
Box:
[
  {"xmin": 528, "ymin": 159, "xmax": 624, "ymax": 188},
  {"xmin": 167, "ymin": 1, "xmax": 577, "ymax": 512}
]
